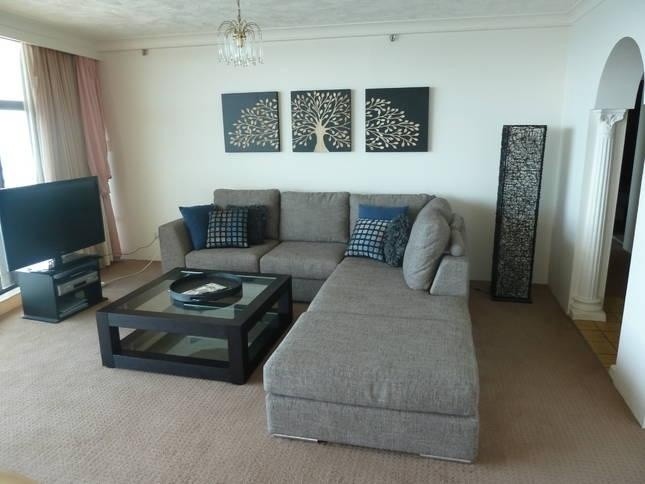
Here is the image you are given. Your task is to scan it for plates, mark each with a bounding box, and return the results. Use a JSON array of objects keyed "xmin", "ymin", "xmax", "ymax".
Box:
[{"xmin": 169, "ymin": 271, "xmax": 243, "ymax": 305}]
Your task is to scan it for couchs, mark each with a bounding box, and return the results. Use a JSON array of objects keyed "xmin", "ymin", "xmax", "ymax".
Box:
[{"xmin": 158, "ymin": 188, "xmax": 484, "ymax": 467}]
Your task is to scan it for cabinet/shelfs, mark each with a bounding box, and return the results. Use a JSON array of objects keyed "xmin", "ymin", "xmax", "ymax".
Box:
[{"xmin": 14, "ymin": 253, "xmax": 108, "ymax": 324}]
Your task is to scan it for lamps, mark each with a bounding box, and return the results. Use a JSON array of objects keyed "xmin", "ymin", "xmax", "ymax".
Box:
[{"xmin": 216, "ymin": 0, "xmax": 266, "ymax": 68}]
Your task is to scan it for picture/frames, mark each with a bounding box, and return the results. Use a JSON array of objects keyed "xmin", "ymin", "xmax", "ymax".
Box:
[
  {"xmin": 290, "ymin": 89, "xmax": 352, "ymax": 152},
  {"xmin": 221, "ymin": 91, "xmax": 280, "ymax": 152},
  {"xmin": 365, "ymin": 87, "xmax": 429, "ymax": 152}
]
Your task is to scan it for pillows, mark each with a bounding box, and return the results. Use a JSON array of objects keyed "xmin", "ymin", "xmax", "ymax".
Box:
[
  {"xmin": 382, "ymin": 213, "xmax": 412, "ymax": 268},
  {"xmin": 179, "ymin": 203, "xmax": 214, "ymax": 250},
  {"xmin": 225, "ymin": 204, "xmax": 268, "ymax": 245},
  {"xmin": 359, "ymin": 203, "xmax": 409, "ymax": 220},
  {"xmin": 205, "ymin": 208, "xmax": 249, "ymax": 249},
  {"xmin": 343, "ymin": 218, "xmax": 392, "ymax": 263}
]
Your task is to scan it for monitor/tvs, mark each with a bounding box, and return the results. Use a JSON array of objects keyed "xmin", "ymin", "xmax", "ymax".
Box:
[{"xmin": 0, "ymin": 176, "xmax": 105, "ymax": 272}]
